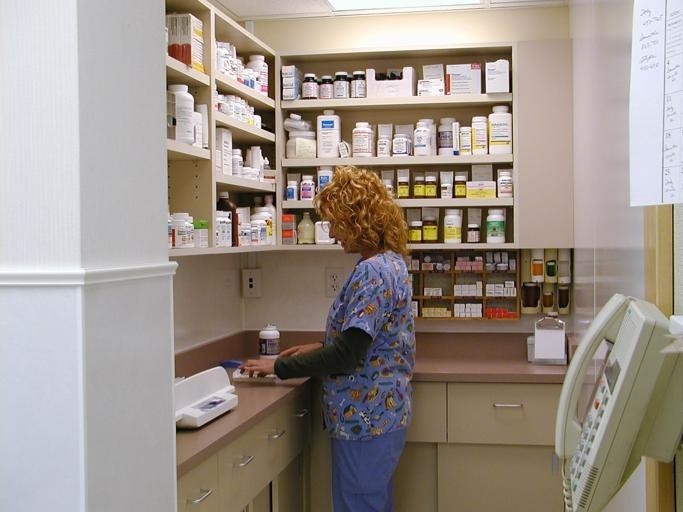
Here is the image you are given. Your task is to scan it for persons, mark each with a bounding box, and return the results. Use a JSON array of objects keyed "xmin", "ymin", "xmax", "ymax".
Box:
[{"xmin": 235, "ymin": 165, "xmax": 417, "ymax": 511}]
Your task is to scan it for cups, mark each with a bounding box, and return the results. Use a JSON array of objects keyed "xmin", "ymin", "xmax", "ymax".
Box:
[{"xmin": 407, "ymin": 208, "xmax": 506, "ymax": 243}]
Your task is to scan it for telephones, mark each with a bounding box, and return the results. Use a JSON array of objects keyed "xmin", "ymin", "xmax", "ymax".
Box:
[{"xmin": 555, "ymin": 293, "xmax": 683, "ymax": 512}]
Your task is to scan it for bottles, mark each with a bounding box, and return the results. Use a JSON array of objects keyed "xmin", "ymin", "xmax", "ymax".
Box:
[
  {"xmin": 301, "ymin": 70, "xmax": 365, "ymax": 98},
  {"xmin": 286, "ymin": 165, "xmax": 333, "ymax": 200},
  {"xmin": 453, "ymin": 174, "xmax": 467, "ymax": 197},
  {"xmin": 413, "ymin": 122, "xmax": 431, "ymax": 156},
  {"xmin": 397, "ymin": 176, "xmax": 437, "ymax": 198},
  {"xmin": 376, "ymin": 135, "xmax": 390, "ymax": 158},
  {"xmin": 257, "ymin": 322, "xmax": 280, "ymax": 360},
  {"xmin": 297, "ymin": 211, "xmax": 315, "ymax": 245},
  {"xmin": 230, "ymin": 147, "xmax": 244, "ymax": 176},
  {"xmin": 166, "ymin": 213, "xmax": 209, "ymax": 249},
  {"xmin": 215, "ymin": 190, "xmax": 238, "ymax": 247},
  {"xmin": 315, "ymin": 108, "xmax": 341, "ymax": 159},
  {"xmin": 437, "ymin": 106, "xmax": 512, "ymax": 155},
  {"xmin": 167, "ymin": 84, "xmax": 194, "ymax": 143},
  {"xmin": 212, "ymin": 83, "xmax": 262, "ymax": 129},
  {"xmin": 351, "ymin": 119, "xmax": 374, "ymax": 158},
  {"xmin": 496, "ymin": 171, "xmax": 512, "ymax": 198},
  {"xmin": 263, "ymin": 155, "xmax": 271, "ymax": 180},
  {"xmin": 250, "ymin": 193, "xmax": 277, "ymax": 245},
  {"xmin": 235, "ymin": 54, "xmax": 269, "ymax": 97}
]
